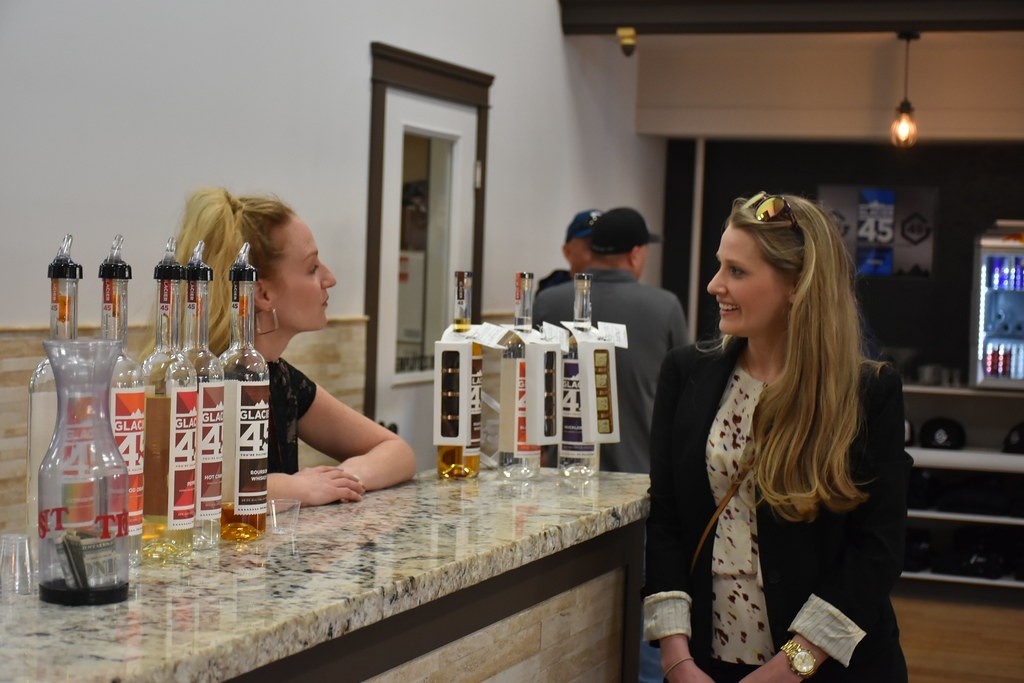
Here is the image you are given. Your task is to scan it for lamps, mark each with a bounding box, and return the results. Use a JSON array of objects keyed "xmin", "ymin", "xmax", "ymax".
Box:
[{"xmin": 888, "ymin": 32, "xmax": 919, "ymax": 145}]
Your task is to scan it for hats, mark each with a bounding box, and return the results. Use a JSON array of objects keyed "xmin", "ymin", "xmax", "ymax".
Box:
[
  {"xmin": 565, "ymin": 208, "xmax": 605, "ymax": 242},
  {"xmin": 588, "ymin": 208, "xmax": 661, "ymax": 253}
]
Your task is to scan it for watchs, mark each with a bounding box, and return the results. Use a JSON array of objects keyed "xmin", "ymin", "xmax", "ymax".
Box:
[{"xmin": 780, "ymin": 640, "xmax": 820, "ymax": 680}]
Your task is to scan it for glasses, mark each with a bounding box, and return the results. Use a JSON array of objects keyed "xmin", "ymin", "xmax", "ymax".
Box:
[{"xmin": 741, "ymin": 190, "xmax": 801, "ymax": 236}]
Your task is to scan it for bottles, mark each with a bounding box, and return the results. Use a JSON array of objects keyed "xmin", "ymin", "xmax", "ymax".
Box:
[
  {"xmin": 218, "ymin": 242, "xmax": 270, "ymax": 543},
  {"xmin": 987, "ymin": 255, "xmax": 1024, "ymax": 381},
  {"xmin": 437, "ymin": 271, "xmax": 483, "ymax": 483},
  {"xmin": 37, "ymin": 340, "xmax": 132, "ymax": 607},
  {"xmin": 556, "ymin": 272, "xmax": 601, "ymax": 487},
  {"xmin": 184, "ymin": 239, "xmax": 224, "ymax": 551},
  {"xmin": 138, "ymin": 237, "xmax": 200, "ymax": 567},
  {"xmin": 27, "ymin": 234, "xmax": 84, "ymax": 591},
  {"xmin": 96, "ymin": 235, "xmax": 146, "ymax": 570},
  {"xmin": 500, "ymin": 272, "xmax": 545, "ymax": 481}
]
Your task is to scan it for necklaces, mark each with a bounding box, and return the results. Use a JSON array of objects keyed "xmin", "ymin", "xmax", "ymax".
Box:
[{"xmin": 743, "ymin": 351, "xmax": 753, "ymax": 378}]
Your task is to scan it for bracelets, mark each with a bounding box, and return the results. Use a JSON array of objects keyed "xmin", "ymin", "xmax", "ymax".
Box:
[{"xmin": 663, "ymin": 656, "xmax": 693, "ymax": 678}]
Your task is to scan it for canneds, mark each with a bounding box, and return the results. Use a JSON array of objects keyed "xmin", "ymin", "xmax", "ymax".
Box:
[{"xmin": 985, "ymin": 342, "xmax": 1022, "ymax": 379}]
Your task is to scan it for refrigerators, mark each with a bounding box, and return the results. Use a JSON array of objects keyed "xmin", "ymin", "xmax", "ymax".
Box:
[{"xmin": 966, "ymin": 236, "xmax": 1024, "ymax": 390}]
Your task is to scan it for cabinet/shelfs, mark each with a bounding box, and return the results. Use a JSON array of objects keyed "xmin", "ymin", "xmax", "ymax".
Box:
[{"xmin": 901, "ymin": 387, "xmax": 1024, "ymax": 586}]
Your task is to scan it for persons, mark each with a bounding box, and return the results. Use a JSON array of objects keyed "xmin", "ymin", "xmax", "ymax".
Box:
[
  {"xmin": 518, "ymin": 206, "xmax": 688, "ymax": 474},
  {"xmin": 638, "ymin": 190, "xmax": 914, "ymax": 683},
  {"xmin": 134, "ymin": 187, "xmax": 415, "ymax": 515}
]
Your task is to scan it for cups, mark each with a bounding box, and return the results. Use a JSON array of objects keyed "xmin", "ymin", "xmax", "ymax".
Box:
[
  {"xmin": 270, "ymin": 499, "xmax": 301, "ymax": 542},
  {"xmin": 1, "ymin": 535, "xmax": 32, "ymax": 604}
]
